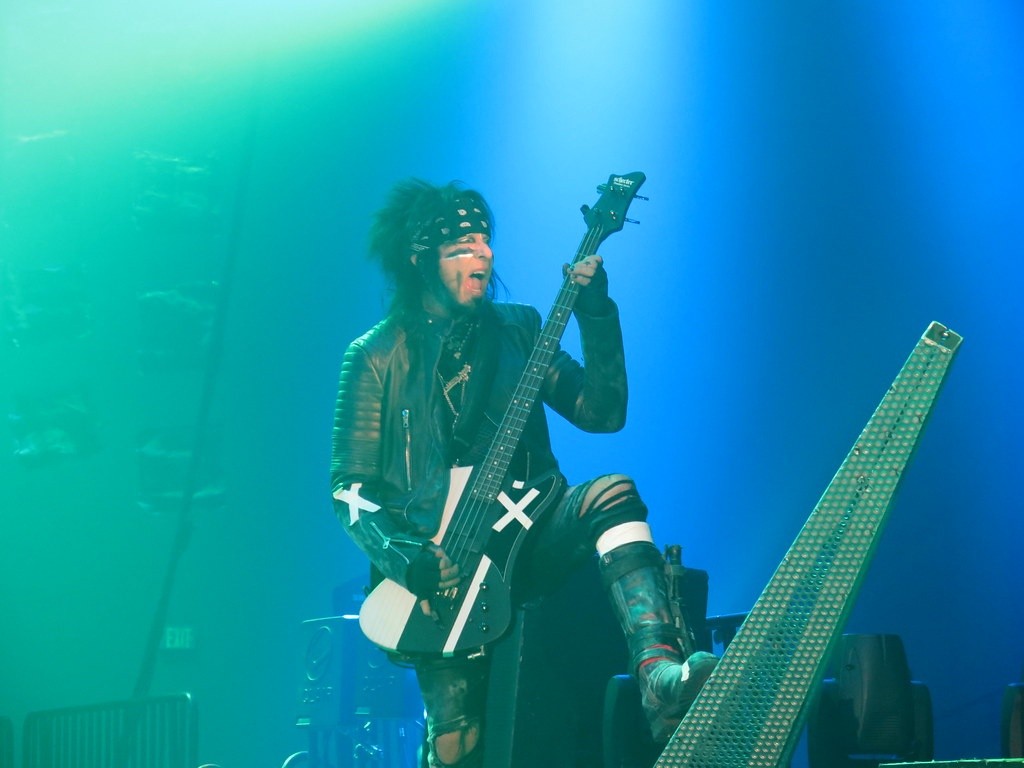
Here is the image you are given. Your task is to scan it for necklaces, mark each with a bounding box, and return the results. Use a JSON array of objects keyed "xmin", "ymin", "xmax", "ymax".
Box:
[{"xmin": 444, "ymin": 320, "xmax": 475, "ymax": 360}]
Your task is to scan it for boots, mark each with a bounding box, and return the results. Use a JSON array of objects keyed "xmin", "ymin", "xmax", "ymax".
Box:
[{"xmin": 597, "ymin": 541, "xmax": 720, "ymax": 745}]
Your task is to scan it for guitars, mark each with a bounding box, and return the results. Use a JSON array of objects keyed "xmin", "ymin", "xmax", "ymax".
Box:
[{"xmin": 357, "ymin": 172, "xmax": 650, "ymax": 658}]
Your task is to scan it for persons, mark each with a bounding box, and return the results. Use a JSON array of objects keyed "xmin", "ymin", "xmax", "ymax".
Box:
[{"xmin": 331, "ymin": 182, "xmax": 724, "ymax": 767}]
